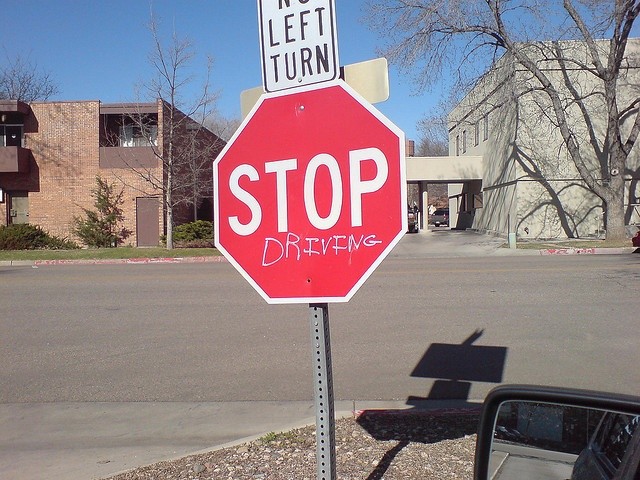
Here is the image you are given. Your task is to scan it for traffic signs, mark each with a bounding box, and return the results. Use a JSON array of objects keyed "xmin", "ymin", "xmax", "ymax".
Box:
[{"xmin": 255, "ymin": 0, "xmax": 341, "ymax": 94}]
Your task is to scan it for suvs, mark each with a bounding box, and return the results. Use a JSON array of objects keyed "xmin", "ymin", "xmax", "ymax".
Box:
[{"xmin": 431, "ymin": 208, "xmax": 449, "ymax": 227}]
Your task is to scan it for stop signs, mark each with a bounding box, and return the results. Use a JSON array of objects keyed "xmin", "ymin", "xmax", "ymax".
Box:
[{"xmin": 214, "ymin": 79, "xmax": 409, "ymax": 304}]
